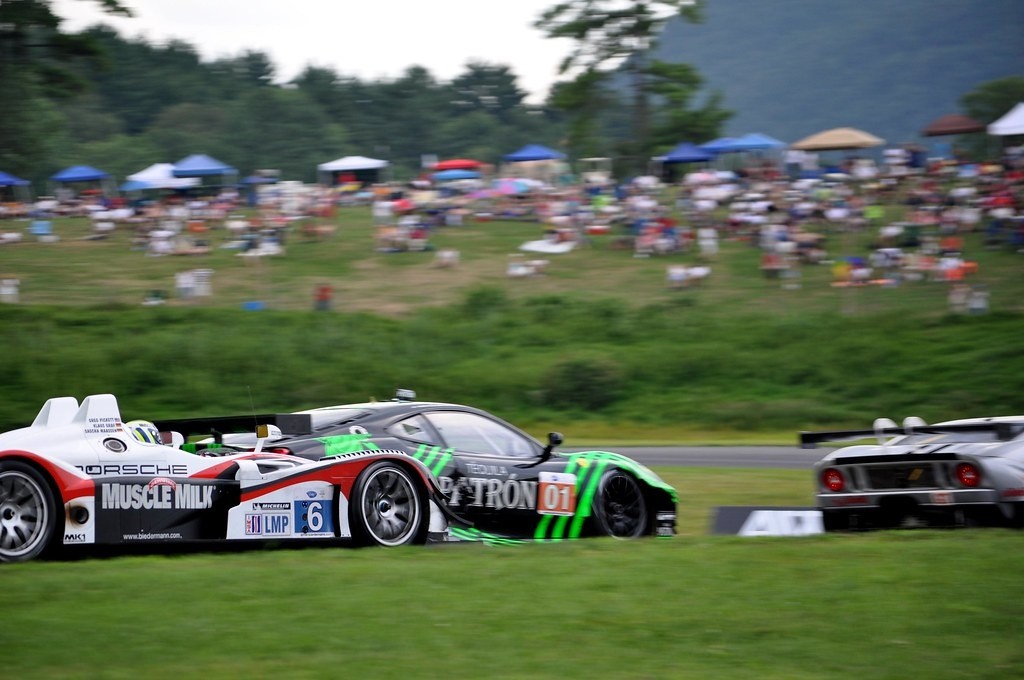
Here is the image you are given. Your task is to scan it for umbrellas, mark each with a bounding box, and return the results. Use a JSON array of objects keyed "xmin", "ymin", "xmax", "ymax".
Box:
[
  {"xmin": 173, "ymin": 154, "xmax": 235, "ymax": 177},
  {"xmin": 652, "ymin": 141, "xmax": 717, "ymax": 173},
  {"xmin": 695, "ymin": 136, "xmax": 751, "ymax": 170},
  {"xmin": 985, "ymin": 102, "xmax": 1024, "ymax": 137},
  {"xmin": 429, "ymin": 169, "xmax": 481, "ymax": 180},
  {"xmin": 0, "ymin": 170, "xmax": 31, "ymax": 186},
  {"xmin": 924, "ymin": 114, "xmax": 985, "ymax": 156},
  {"xmin": 501, "ymin": 143, "xmax": 567, "ymax": 161},
  {"xmin": 239, "ymin": 175, "xmax": 276, "ymax": 184},
  {"xmin": 317, "ymin": 155, "xmax": 389, "ymax": 170},
  {"xmin": 739, "ymin": 133, "xmax": 789, "ymax": 168},
  {"xmin": 49, "ymin": 164, "xmax": 110, "ymax": 181},
  {"xmin": 428, "ymin": 158, "xmax": 487, "ymax": 169},
  {"xmin": 124, "ymin": 163, "xmax": 203, "ymax": 187},
  {"xmin": 0, "ymin": 144, "xmax": 1023, "ymax": 308},
  {"xmin": 788, "ymin": 127, "xmax": 886, "ymax": 169},
  {"xmin": 116, "ymin": 180, "xmax": 151, "ymax": 191}
]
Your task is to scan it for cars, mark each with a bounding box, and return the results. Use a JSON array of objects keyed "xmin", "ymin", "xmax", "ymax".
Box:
[
  {"xmin": 800, "ymin": 394, "xmax": 1024, "ymax": 531},
  {"xmin": 0, "ymin": 389, "xmax": 679, "ymax": 565}
]
